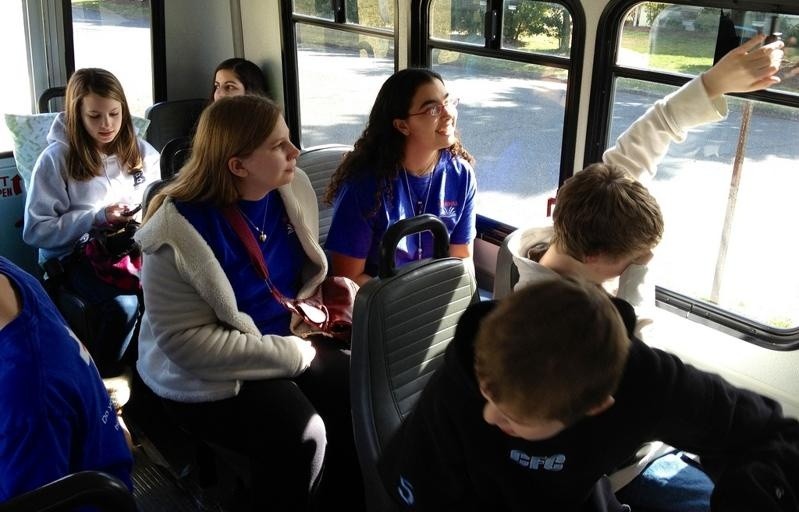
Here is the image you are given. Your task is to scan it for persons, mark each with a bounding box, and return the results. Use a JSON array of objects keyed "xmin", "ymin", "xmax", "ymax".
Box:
[
  {"xmin": 493, "ymin": 33, "xmax": 785, "ymax": 338},
  {"xmin": 24, "ymin": 66, "xmax": 162, "ymax": 372},
  {"xmin": 1, "ymin": 250, "xmax": 135, "ymax": 504},
  {"xmin": 209, "ymin": 57, "xmax": 274, "ymax": 101},
  {"xmin": 323, "ymin": 69, "xmax": 478, "ymax": 302},
  {"xmin": 376, "ymin": 281, "xmax": 797, "ymax": 512},
  {"xmin": 138, "ymin": 95, "xmax": 354, "ymax": 512}
]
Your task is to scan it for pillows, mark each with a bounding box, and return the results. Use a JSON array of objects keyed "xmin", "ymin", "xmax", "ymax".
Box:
[{"xmin": 4, "ymin": 114, "xmax": 151, "ymax": 194}]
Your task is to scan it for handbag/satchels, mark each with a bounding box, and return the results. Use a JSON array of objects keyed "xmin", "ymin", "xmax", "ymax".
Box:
[
  {"xmin": 226, "ymin": 205, "xmax": 361, "ymax": 351},
  {"xmin": 85, "ymin": 233, "xmax": 146, "ymax": 292}
]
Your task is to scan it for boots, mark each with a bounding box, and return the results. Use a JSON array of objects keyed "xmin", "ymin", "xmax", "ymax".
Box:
[{"xmin": 96, "ymin": 377, "xmax": 132, "ymax": 454}]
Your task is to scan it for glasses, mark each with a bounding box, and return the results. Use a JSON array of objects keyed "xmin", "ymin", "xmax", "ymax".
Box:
[{"xmin": 400, "ymin": 98, "xmax": 461, "ymax": 115}]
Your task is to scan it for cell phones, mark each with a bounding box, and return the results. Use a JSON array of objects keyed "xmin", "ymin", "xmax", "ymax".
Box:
[{"xmin": 120, "ymin": 202, "xmax": 141, "ymax": 217}]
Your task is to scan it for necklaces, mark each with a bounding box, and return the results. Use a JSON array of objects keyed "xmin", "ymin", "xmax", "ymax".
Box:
[
  {"xmin": 232, "ymin": 194, "xmax": 271, "ymax": 242},
  {"xmin": 396, "ymin": 159, "xmax": 442, "ymax": 259}
]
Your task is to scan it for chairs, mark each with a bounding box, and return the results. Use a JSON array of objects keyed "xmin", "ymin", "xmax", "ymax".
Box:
[
  {"xmin": 133, "ymin": 439, "xmax": 213, "ymax": 511},
  {"xmin": 117, "ymin": 135, "xmax": 263, "ymax": 496},
  {"xmin": 145, "ymin": 97, "xmax": 212, "ymax": 172},
  {"xmin": 297, "ymin": 142, "xmax": 354, "ymax": 247},
  {"xmin": 349, "ymin": 217, "xmax": 481, "ymax": 471},
  {"xmin": 1, "ymin": 469, "xmax": 138, "ymax": 511},
  {"xmin": 493, "ymin": 226, "xmax": 519, "ymax": 299},
  {"xmin": 39, "ymin": 86, "xmax": 87, "ymax": 314}
]
